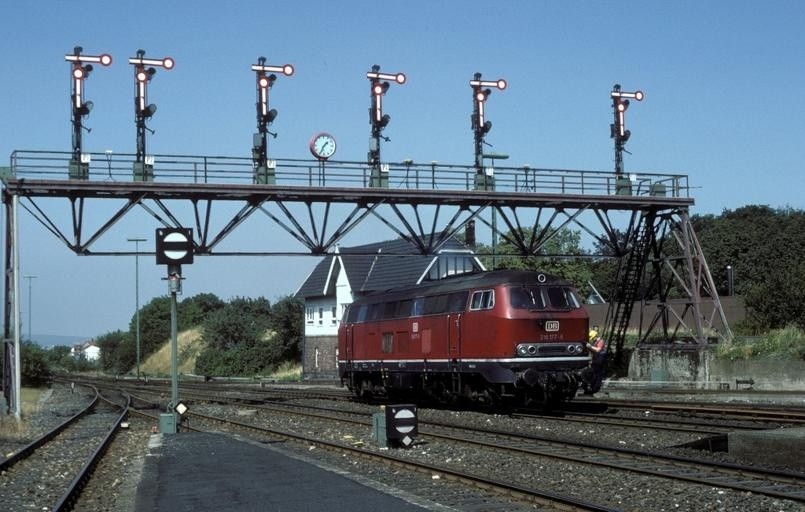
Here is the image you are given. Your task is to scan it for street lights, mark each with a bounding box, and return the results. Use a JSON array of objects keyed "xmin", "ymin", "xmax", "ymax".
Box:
[
  {"xmin": 22, "ymin": 274, "xmax": 39, "ymax": 342},
  {"xmin": 126, "ymin": 238, "xmax": 148, "ymax": 382},
  {"xmin": 726, "ymin": 264, "xmax": 734, "ymax": 296}
]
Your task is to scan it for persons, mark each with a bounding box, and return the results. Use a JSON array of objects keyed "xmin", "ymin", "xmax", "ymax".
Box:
[{"xmin": 586, "ymin": 330, "xmax": 607, "ymax": 393}]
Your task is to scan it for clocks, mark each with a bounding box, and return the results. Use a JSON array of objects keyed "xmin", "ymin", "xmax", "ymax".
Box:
[{"xmin": 309, "ymin": 132, "xmax": 337, "ymax": 159}]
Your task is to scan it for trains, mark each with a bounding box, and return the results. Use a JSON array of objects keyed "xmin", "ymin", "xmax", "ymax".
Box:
[{"xmin": 334, "ymin": 268, "xmax": 590, "ymax": 408}]
